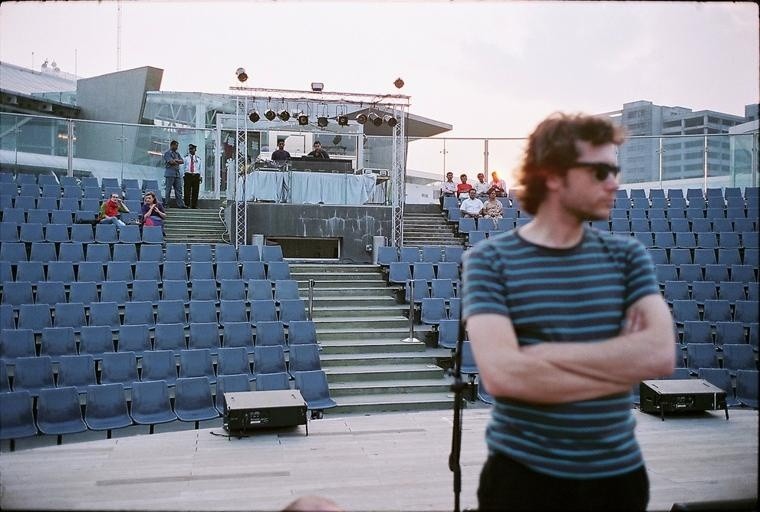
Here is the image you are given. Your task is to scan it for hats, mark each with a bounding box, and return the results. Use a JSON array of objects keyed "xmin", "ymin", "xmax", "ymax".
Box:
[{"xmin": 189, "ymin": 144, "xmax": 197, "ymax": 148}]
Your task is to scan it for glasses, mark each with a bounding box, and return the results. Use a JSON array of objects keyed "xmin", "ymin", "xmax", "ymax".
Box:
[{"xmin": 555, "ymin": 161, "xmax": 622, "ymax": 181}]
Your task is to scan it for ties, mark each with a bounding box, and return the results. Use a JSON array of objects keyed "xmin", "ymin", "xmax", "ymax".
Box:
[{"xmin": 190, "ymin": 155, "xmax": 195, "ymax": 173}]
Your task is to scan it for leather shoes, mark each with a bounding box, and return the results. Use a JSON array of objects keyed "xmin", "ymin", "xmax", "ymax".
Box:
[
  {"xmin": 163, "ymin": 204, "xmax": 169, "ymax": 208},
  {"xmin": 181, "ymin": 204, "xmax": 199, "ymax": 209}
]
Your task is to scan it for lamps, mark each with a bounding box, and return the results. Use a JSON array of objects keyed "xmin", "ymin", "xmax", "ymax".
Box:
[
  {"xmin": 310, "ymin": 82, "xmax": 324, "ymax": 92},
  {"xmin": 246, "ymin": 95, "xmax": 397, "ymax": 131},
  {"xmin": 235, "ymin": 66, "xmax": 248, "ymax": 89}
]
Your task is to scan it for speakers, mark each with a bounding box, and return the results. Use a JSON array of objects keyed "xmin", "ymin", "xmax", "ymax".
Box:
[
  {"xmin": 251, "ymin": 234, "xmax": 264, "ymax": 259},
  {"xmin": 641, "ymin": 379, "xmax": 729, "ymax": 419},
  {"xmin": 372, "ymin": 236, "xmax": 386, "ymax": 264},
  {"xmin": 223, "ymin": 389, "xmax": 307, "ymax": 432}
]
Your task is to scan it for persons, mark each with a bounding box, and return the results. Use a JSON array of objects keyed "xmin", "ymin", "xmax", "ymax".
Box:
[
  {"xmin": 98, "ymin": 193, "xmax": 129, "ymax": 226},
  {"xmin": 163, "ymin": 141, "xmax": 188, "ymax": 208},
  {"xmin": 309, "ymin": 141, "xmax": 329, "ymax": 157},
  {"xmin": 142, "ymin": 192, "xmax": 167, "ymax": 222},
  {"xmin": 441, "ymin": 172, "xmax": 509, "ymax": 224},
  {"xmin": 183, "ymin": 144, "xmax": 202, "ymax": 209},
  {"xmin": 461, "ymin": 114, "xmax": 677, "ymax": 511},
  {"xmin": 272, "ymin": 139, "xmax": 290, "ymax": 160}
]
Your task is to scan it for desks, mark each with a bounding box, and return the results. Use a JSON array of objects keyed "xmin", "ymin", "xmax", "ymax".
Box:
[{"xmin": 237, "ymin": 167, "xmax": 390, "ymax": 205}]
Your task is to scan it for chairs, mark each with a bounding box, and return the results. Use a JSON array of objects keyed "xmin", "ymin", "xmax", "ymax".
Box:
[
  {"xmin": 1, "ymin": 163, "xmax": 333, "ymax": 460},
  {"xmin": 375, "ymin": 182, "xmax": 758, "ymax": 419}
]
